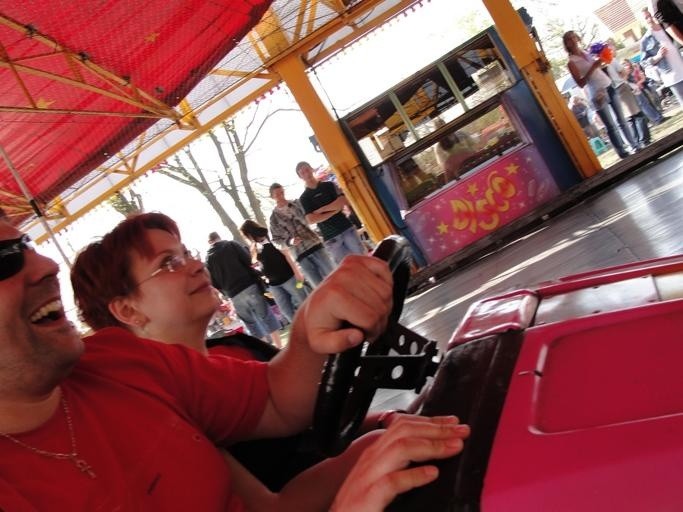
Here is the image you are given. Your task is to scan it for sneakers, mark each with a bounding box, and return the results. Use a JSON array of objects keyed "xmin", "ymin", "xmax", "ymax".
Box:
[{"xmin": 620, "ymin": 115, "xmax": 671, "ymax": 158}]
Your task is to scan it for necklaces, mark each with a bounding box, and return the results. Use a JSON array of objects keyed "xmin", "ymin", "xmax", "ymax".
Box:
[{"xmin": 0, "ymin": 382, "xmax": 97, "ymax": 479}]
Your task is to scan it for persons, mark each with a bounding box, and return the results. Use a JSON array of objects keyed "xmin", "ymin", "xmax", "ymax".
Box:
[
  {"xmin": 1, "ymin": 209, "xmax": 472, "ymax": 509},
  {"xmin": 384, "ymin": 128, "xmax": 436, "ymax": 192},
  {"xmin": 431, "ymin": 116, "xmax": 484, "ymax": 173},
  {"xmin": 68, "ymin": 211, "xmax": 435, "ymax": 494},
  {"xmin": 564, "ymin": 1, "xmax": 683, "ymax": 161},
  {"xmin": 204, "ymin": 161, "xmax": 370, "ymax": 351}
]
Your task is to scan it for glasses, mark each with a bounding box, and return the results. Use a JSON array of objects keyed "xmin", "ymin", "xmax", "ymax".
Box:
[
  {"xmin": 120, "ymin": 246, "xmax": 202, "ymax": 291},
  {"xmin": 0, "ymin": 233, "xmax": 37, "ymax": 281}
]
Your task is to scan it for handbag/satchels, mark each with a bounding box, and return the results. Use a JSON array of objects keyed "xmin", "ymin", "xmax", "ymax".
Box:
[{"xmin": 587, "ymin": 86, "xmax": 610, "ymax": 112}]
